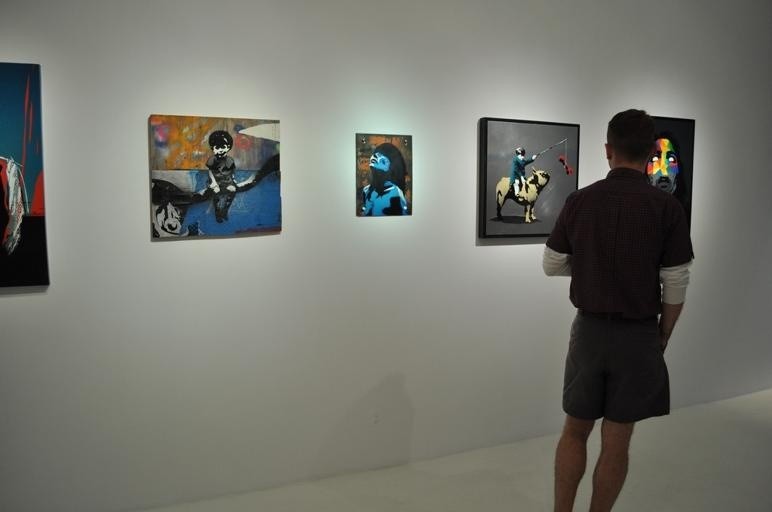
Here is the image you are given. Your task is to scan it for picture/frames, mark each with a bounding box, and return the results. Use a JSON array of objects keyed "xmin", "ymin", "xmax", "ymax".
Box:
[{"xmin": 477, "ymin": 118, "xmax": 580, "ymax": 238}]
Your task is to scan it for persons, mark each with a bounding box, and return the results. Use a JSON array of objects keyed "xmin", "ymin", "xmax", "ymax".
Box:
[
  {"xmin": 539, "ymin": 109, "xmax": 695, "ymax": 512},
  {"xmin": 359, "ymin": 142, "xmax": 410, "ymax": 216}
]
